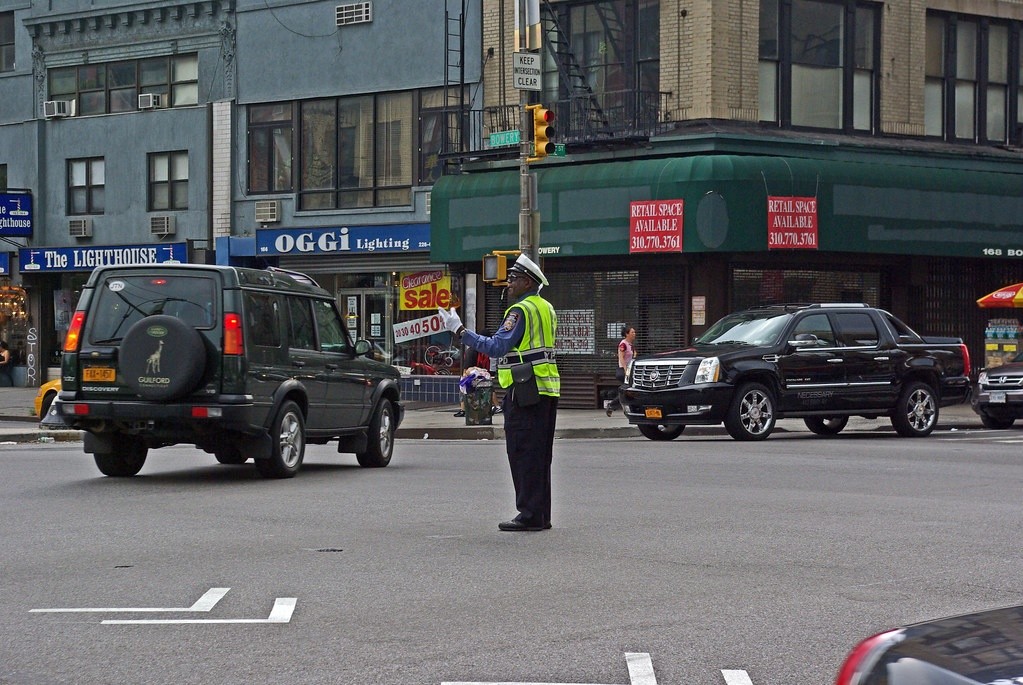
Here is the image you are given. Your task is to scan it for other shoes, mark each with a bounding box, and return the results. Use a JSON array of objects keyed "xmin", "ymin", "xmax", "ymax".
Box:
[
  {"xmin": 492, "ymin": 405, "xmax": 503, "ymax": 413},
  {"xmin": 454, "ymin": 409, "xmax": 466, "ymax": 417},
  {"xmin": 606, "ymin": 403, "xmax": 613, "ymax": 417},
  {"xmin": 498, "ymin": 513, "xmax": 552, "ymax": 530}
]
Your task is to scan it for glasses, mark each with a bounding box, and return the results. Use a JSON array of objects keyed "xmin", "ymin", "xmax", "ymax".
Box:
[{"xmin": 508, "ymin": 274, "xmax": 530, "ymax": 281}]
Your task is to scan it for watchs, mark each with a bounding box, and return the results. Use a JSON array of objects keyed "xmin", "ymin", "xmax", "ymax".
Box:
[{"xmin": 458, "ymin": 329, "xmax": 467, "ymax": 339}]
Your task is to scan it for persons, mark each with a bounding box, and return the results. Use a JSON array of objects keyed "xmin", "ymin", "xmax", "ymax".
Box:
[
  {"xmin": 453, "ymin": 329, "xmax": 502, "ymax": 417},
  {"xmin": 0, "ymin": 341, "xmax": 12, "ymax": 376},
  {"xmin": 606, "ymin": 325, "xmax": 638, "ymax": 417},
  {"xmin": 438, "ymin": 254, "xmax": 561, "ymax": 531}
]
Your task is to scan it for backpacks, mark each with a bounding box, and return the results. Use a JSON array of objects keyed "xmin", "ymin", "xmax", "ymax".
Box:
[{"xmin": 477, "ymin": 352, "xmax": 497, "ymax": 378}]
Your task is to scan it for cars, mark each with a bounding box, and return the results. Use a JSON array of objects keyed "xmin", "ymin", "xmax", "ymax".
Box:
[
  {"xmin": 34, "ymin": 378, "xmax": 62, "ymax": 421},
  {"xmin": 367, "ymin": 338, "xmax": 460, "ymax": 375},
  {"xmin": 835, "ymin": 605, "xmax": 1023, "ymax": 684},
  {"xmin": 971, "ymin": 350, "xmax": 1023, "ymax": 429}
]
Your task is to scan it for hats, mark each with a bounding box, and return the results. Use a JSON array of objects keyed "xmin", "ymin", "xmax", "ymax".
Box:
[{"xmin": 507, "ymin": 254, "xmax": 550, "ymax": 286}]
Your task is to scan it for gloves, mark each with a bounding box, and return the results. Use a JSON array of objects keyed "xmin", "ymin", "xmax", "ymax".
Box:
[{"xmin": 438, "ymin": 306, "xmax": 463, "ymax": 335}]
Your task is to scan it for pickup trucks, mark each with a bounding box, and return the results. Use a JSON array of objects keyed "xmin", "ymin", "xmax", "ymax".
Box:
[{"xmin": 617, "ymin": 308, "xmax": 973, "ymax": 441}]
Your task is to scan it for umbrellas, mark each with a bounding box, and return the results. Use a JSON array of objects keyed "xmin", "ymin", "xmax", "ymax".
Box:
[{"xmin": 976, "ymin": 282, "xmax": 1023, "ymax": 308}]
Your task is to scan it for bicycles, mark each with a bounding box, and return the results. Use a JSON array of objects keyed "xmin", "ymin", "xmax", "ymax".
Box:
[{"xmin": 424, "ymin": 344, "xmax": 458, "ymax": 375}]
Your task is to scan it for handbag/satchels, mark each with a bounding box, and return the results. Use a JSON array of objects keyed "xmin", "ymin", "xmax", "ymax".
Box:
[
  {"xmin": 616, "ymin": 367, "xmax": 624, "ymax": 382},
  {"xmin": 511, "ymin": 362, "xmax": 540, "ymax": 407}
]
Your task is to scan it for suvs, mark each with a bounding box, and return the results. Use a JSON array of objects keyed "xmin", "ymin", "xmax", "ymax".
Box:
[{"xmin": 54, "ymin": 259, "xmax": 406, "ymax": 477}]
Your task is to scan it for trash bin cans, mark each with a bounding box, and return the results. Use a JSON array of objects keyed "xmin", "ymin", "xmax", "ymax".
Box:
[{"xmin": 462, "ymin": 380, "xmax": 493, "ymax": 426}]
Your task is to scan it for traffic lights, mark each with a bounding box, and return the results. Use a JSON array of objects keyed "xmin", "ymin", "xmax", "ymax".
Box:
[
  {"xmin": 534, "ymin": 108, "xmax": 557, "ymax": 159},
  {"xmin": 482, "ymin": 255, "xmax": 507, "ymax": 282}
]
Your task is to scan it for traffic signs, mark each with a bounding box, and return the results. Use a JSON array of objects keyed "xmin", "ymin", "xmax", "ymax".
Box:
[{"xmin": 528, "ymin": 143, "xmax": 565, "ymax": 157}]
